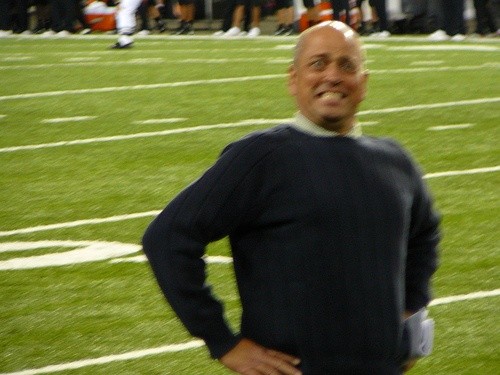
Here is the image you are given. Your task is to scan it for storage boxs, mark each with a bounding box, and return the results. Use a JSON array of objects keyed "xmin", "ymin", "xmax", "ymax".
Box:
[{"xmin": 84, "ymin": 13, "xmax": 115, "ymax": 30}]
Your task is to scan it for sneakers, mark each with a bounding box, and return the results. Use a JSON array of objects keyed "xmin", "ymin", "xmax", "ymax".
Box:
[
  {"xmin": 1, "ymin": 27, "xmax": 91, "ymax": 37},
  {"xmin": 352, "ymin": 27, "xmax": 500, "ymax": 42},
  {"xmin": 116, "ymin": 34, "xmax": 134, "ymax": 47},
  {"xmin": 274, "ymin": 24, "xmax": 298, "ymax": 36},
  {"xmin": 137, "ymin": 29, "xmax": 152, "ymax": 36},
  {"xmin": 212, "ymin": 26, "xmax": 260, "ymax": 38}
]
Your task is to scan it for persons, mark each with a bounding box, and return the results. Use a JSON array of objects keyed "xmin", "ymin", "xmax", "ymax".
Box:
[
  {"xmin": 0, "ymin": 0, "xmax": 500, "ymax": 51},
  {"xmin": 142, "ymin": 20, "xmax": 441, "ymax": 375}
]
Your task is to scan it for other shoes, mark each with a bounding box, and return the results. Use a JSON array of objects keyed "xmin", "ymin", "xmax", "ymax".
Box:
[
  {"xmin": 149, "ymin": 20, "xmax": 166, "ymax": 34},
  {"xmin": 169, "ymin": 22, "xmax": 194, "ymax": 36}
]
